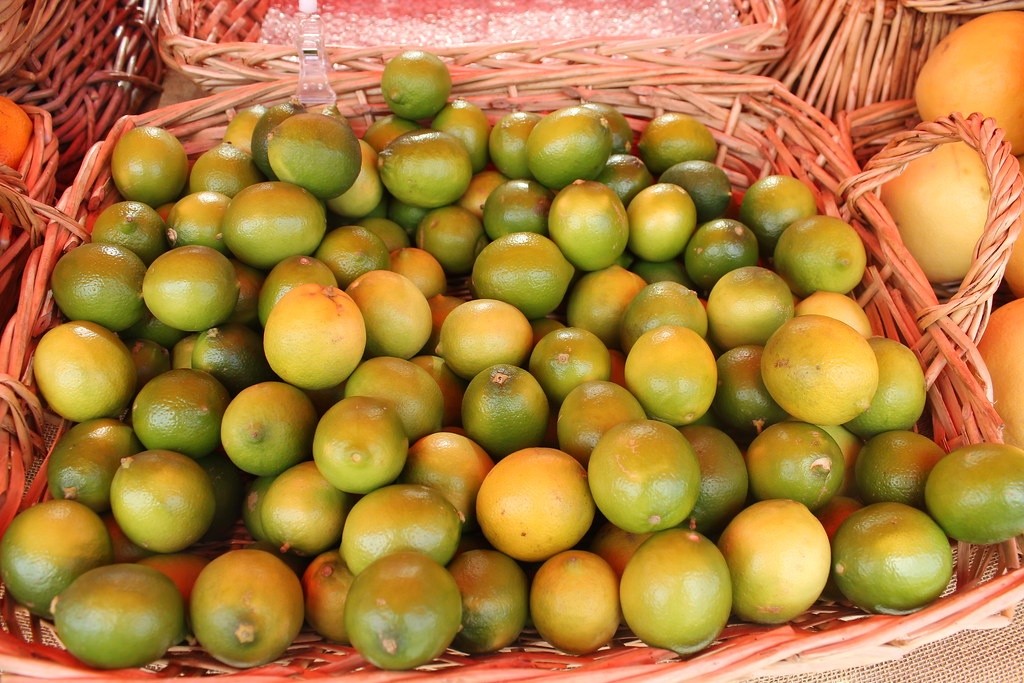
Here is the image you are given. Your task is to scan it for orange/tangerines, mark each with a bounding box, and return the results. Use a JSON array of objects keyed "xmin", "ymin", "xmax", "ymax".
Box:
[{"xmin": 0, "ymin": 52, "xmax": 1024, "ymax": 671}]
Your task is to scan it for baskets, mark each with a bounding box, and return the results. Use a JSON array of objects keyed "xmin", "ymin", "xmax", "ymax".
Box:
[
  {"xmin": 0, "ymin": 0, "xmax": 165, "ymax": 191},
  {"xmin": 0, "ymin": 105, "xmax": 60, "ymax": 338},
  {"xmin": 763, "ymin": 0, "xmax": 1024, "ymax": 113},
  {"xmin": 0, "ymin": 59, "xmax": 1024, "ymax": 683},
  {"xmin": 156, "ymin": 0, "xmax": 789, "ymax": 105}
]
